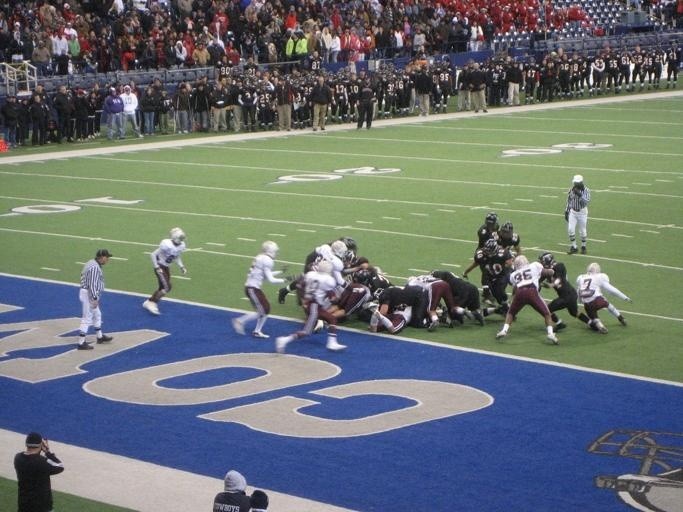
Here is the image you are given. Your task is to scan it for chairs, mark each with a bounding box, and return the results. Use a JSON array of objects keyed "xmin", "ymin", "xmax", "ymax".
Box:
[{"xmin": 1, "ymin": 0, "xmax": 682, "ymax": 144}]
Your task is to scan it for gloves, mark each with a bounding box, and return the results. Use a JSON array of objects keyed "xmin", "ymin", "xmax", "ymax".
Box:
[
  {"xmin": 573, "ymin": 187, "xmax": 583, "ymax": 197},
  {"xmin": 564, "ymin": 211, "xmax": 569, "ymax": 222},
  {"xmin": 180, "ymin": 267, "xmax": 186, "ymax": 275},
  {"xmin": 154, "ymin": 266, "xmax": 163, "ymax": 276}
]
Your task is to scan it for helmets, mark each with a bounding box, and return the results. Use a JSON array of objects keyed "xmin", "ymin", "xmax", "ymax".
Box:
[
  {"xmin": 513, "ymin": 255, "xmax": 529, "ymax": 269},
  {"xmin": 482, "ymin": 239, "xmax": 500, "ymax": 256},
  {"xmin": 587, "ymin": 262, "xmax": 601, "ymax": 274},
  {"xmin": 539, "ymin": 253, "xmax": 553, "ymax": 266},
  {"xmin": 317, "ymin": 237, "xmax": 385, "ymax": 299},
  {"xmin": 168, "ymin": 227, "xmax": 186, "ymax": 239},
  {"xmin": 485, "ymin": 211, "xmax": 497, "ymax": 224},
  {"xmin": 500, "ymin": 220, "xmax": 513, "ymax": 233},
  {"xmin": 261, "ymin": 240, "xmax": 279, "ymax": 253}
]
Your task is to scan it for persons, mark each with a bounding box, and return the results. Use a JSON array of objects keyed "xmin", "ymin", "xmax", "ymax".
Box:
[
  {"xmin": 564, "ymin": 174, "xmax": 591, "ymax": 255},
  {"xmin": 212, "ymin": 469, "xmax": 251, "ymax": 511},
  {"xmin": 577, "ymin": 262, "xmax": 633, "ymax": 333},
  {"xmin": 495, "ymin": 255, "xmax": 559, "ymax": 345},
  {"xmin": 142, "ymin": 227, "xmax": 188, "ymax": 315},
  {"xmin": 76, "ymin": 249, "xmax": 113, "ymax": 350},
  {"xmin": 13, "ymin": 431, "xmax": 64, "ymax": 512},
  {"xmin": 249, "ymin": 490, "xmax": 269, "ymax": 512},
  {"xmin": 538, "ymin": 252, "xmax": 599, "ymax": 331}
]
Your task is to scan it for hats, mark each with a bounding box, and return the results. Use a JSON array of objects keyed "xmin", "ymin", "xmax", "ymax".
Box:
[
  {"xmin": 96, "ymin": 249, "xmax": 112, "ymax": 257},
  {"xmin": 223, "ymin": 470, "xmax": 246, "ymax": 493},
  {"xmin": 25, "ymin": 431, "xmax": 43, "ymax": 448},
  {"xmin": 572, "ymin": 175, "xmax": 583, "ymax": 183}
]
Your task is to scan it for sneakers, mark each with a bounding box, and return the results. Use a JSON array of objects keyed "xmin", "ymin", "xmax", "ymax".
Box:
[
  {"xmin": 251, "ymin": 329, "xmax": 269, "ymax": 338},
  {"xmin": 553, "ymin": 324, "xmax": 566, "ymax": 331},
  {"xmin": 581, "ymin": 246, "xmax": 587, "ymax": 254},
  {"xmin": 592, "ymin": 319, "xmax": 608, "ymax": 333},
  {"xmin": 326, "ymin": 341, "xmax": 346, "ymax": 350},
  {"xmin": 278, "ymin": 288, "xmax": 284, "ymax": 304},
  {"xmin": 623, "ymin": 318, "xmax": 627, "ymax": 324},
  {"xmin": 275, "ymin": 336, "xmax": 285, "ymax": 355},
  {"xmin": 96, "ymin": 334, "xmax": 111, "ymax": 343},
  {"xmin": 230, "ymin": 317, "xmax": 247, "ymax": 336},
  {"xmin": 427, "ymin": 291, "xmax": 491, "ymax": 330},
  {"xmin": 76, "ymin": 340, "xmax": 93, "ymax": 350},
  {"xmin": 566, "ymin": 245, "xmax": 578, "ymax": 254},
  {"xmin": 547, "ymin": 334, "xmax": 559, "ymax": 344},
  {"xmin": 362, "ymin": 300, "xmax": 379, "ymax": 310},
  {"xmin": 496, "ymin": 332, "xmax": 507, "ymax": 338},
  {"xmin": 312, "ymin": 318, "xmax": 324, "ymax": 334},
  {"xmin": 142, "ymin": 299, "xmax": 161, "ymax": 315}
]
